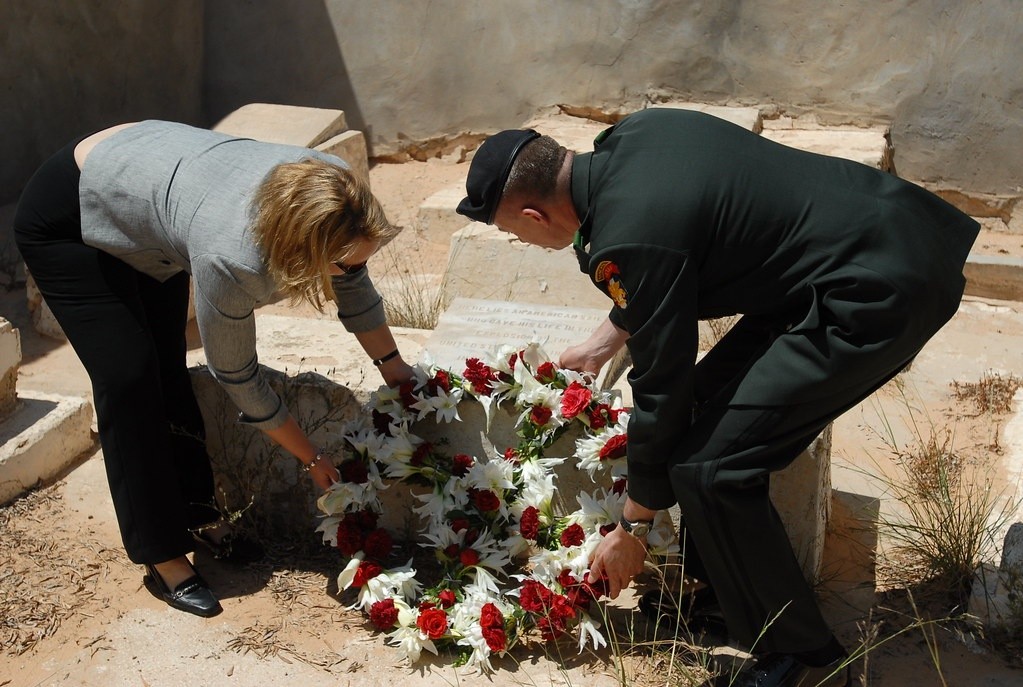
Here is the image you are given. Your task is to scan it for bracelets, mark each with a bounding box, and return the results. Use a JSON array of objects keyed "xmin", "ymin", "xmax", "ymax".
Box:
[
  {"xmin": 301, "ymin": 450, "xmax": 325, "ymax": 473},
  {"xmin": 373, "ymin": 349, "xmax": 399, "ymax": 365}
]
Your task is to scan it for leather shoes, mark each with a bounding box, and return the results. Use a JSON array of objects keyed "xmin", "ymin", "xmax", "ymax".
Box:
[
  {"xmin": 638, "ymin": 590, "xmax": 732, "ymax": 645},
  {"xmin": 698, "ymin": 650, "xmax": 851, "ymax": 687}
]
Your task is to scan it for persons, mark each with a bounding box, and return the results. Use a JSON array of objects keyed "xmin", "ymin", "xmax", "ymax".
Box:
[
  {"xmin": 13, "ymin": 119, "xmax": 418, "ymax": 618},
  {"xmin": 456, "ymin": 108, "xmax": 980, "ymax": 687}
]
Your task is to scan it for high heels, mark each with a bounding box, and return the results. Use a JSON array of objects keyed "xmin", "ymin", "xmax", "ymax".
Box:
[
  {"xmin": 145, "ymin": 553, "xmax": 222, "ymax": 617},
  {"xmin": 198, "ymin": 518, "xmax": 251, "ymax": 568}
]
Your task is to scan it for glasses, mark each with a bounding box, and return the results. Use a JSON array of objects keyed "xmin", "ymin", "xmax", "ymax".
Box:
[{"xmin": 335, "ymin": 262, "xmax": 367, "ymax": 275}]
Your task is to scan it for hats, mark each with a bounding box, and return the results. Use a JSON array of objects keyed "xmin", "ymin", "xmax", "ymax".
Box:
[{"xmin": 456, "ymin": 128, "xmax": 541, "ymax": 225}]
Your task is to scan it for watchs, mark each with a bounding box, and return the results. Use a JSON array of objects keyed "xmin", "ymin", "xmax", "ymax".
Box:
[{"xmin": 620, "ymin": 514, "xmax": 655, "ymax": 539}]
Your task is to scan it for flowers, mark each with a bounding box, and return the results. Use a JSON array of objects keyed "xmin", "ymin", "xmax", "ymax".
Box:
[{"xmin": 313, "ymin": 344, "xmax": 685, "ymax": 680}]
